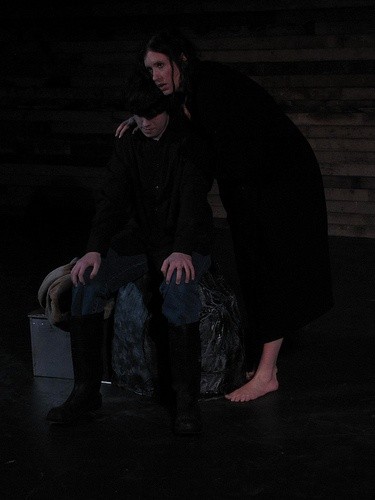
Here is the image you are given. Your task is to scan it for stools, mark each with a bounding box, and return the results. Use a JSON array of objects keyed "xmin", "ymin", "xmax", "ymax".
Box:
[
  {"xmin": 28, "ymin": 308, "xmax": 98, "ymax": 380},
  {"xmin": 111, "ymin": 262, "xmax": 245, "ymax": 400}
]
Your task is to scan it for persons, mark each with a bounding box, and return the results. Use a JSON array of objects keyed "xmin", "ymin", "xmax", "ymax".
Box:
[
  {"xmin": 112, "ymin": 21, "xmax": 329, "ymax": 404},
  {"xmin": 42, "ymin": 88, "xmax": 222, "ymax": 437}
]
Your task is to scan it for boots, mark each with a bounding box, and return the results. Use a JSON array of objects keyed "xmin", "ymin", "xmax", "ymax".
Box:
[
  {"xmin": 160, "ymin": 325, "xmax": 203, "ymax": 440},
  {"xmin": 46, "ymin": 324, "xmax": 102, "ymax": 421}
]
